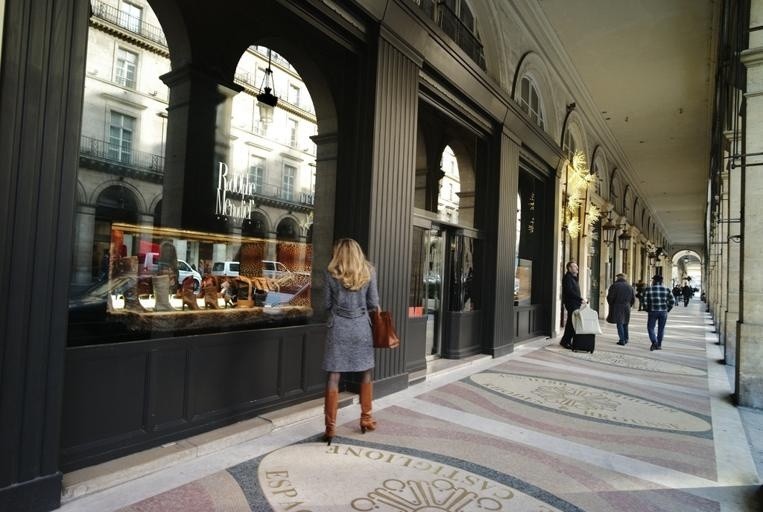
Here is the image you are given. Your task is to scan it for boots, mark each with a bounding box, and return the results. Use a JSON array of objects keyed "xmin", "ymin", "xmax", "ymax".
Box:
[
  {"xmin": 321, "ymin": 388, "xmax": 340, "ymax": 446},
  {"xmin": 355, "ymin": 379, "xmax": 377, "ymax": 434}
]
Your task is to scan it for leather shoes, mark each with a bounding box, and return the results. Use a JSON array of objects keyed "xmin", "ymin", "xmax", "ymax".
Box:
[{"xmin": 560, "ymin": 339, "xmax": 573, "ymax": 349}]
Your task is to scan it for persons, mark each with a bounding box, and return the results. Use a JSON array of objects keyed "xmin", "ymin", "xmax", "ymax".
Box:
[
  {"xmin": 634, "ymin": 279, "xmax": 649, "ymax": 312},
  {"xmin": 155, "ymin": 242, "xmax": 181, "ymax": 295},
  {"xmin": 605, "ymin": 272, "xmax": 636, "ymax": 346},
  {"xmin": 319, "ymin": 236, "xmax": 382, "ymax": 446},
  {"xmin": 198, "ymin": 258, "xmax": 204, "ymax": 276},
  {"xmin": 202, "ymin": 259, "xmax": 212, "ymax": 278},
  {"xmin": 671, "ymin": 282, "xmax": 699, "ymax": 308},
  {"xmin": 643, "ymin": 274, "xmax": 676, "ymax": 351},
  {"xmin": 557, "ymin": 260, "xmax": 588, "ymax": 350}
]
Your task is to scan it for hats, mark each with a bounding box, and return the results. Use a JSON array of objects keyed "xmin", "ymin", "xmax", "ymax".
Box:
[
  {"xmin": 651, "ymin": 274, "xmax": 664, "ymax": 282},
  {"xmin": 616, "ymin": 272, "xmax": 627, "ymax": 278}
]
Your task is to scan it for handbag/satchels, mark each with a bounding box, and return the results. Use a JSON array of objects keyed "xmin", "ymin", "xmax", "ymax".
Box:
[
  {"xmin": 368, "ymin": 302, "xmax": 402, "ymax": 350},
  {"xmin": 571, "ymin": 300, "xmax": 603, "ymax": 336}
]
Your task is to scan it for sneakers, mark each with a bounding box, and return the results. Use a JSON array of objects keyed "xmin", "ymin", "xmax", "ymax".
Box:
[
  {"xmin": 617, "ymin": 337, "xmax": 628, "ymax": 346},
  {"xmin": 649, "ymin": 341, "xmax": 662, "ymax": 351}
]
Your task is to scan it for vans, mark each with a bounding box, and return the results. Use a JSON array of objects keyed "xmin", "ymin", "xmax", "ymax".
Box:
[
  {"xmin": 211, "ymin": 260, "xmax": 241, "ymax": 279},
  {"xmin": 261, "ymin": 260, "xmax": 294, "ymax": 284},
  {"xmin": 133, "ymin": 250, "xmax": 200, "ymax": 294}
]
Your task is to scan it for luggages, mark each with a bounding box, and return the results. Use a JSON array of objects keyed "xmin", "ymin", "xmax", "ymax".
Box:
[{"xmin": 571, "ymin": 327, "xmax": 595, "ymax": 354}]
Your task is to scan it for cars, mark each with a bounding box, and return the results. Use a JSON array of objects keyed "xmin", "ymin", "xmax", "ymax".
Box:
[{"xmin": 421, "ymin": 274, "xmax": 440, "ymax": 283}]
